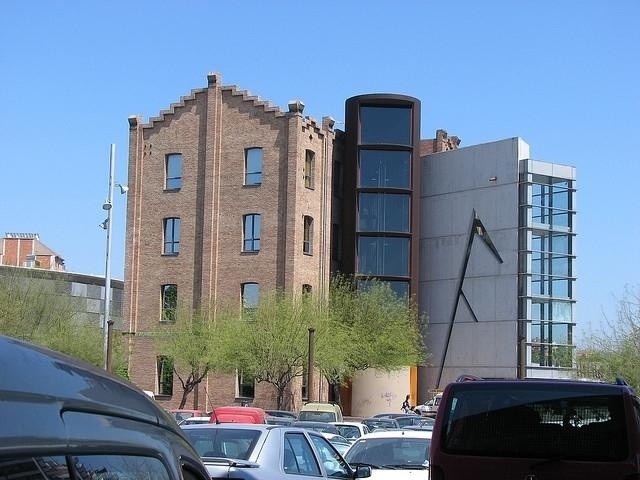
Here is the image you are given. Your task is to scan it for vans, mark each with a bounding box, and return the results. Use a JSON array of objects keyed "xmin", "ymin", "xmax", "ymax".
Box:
[{"xmin": 427, "ymin": 374, "xmax": 639, "ymax": 478}]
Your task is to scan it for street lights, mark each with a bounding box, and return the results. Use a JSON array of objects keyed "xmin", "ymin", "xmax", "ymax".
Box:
[{"xmin": 98, "ymin": 143, "xmax": 130, "ymax": 372}]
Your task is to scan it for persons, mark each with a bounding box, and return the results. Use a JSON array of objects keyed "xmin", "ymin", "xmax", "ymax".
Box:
[
  {"xmin": 403, "ymin": 394, "xmax": 410, "ymax": 413},
  {"xmin": 408, "ymin": 406, "xmax": 416, "ymax": 414}
]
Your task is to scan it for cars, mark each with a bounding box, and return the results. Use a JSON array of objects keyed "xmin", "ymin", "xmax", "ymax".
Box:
[
  {"xmin": 167, "ymin": 394, "xmax": 442, "ymax": 479},
  {"xmin": 1, "ymin": 334, "xmax": 211, "ymax": 478}
]
